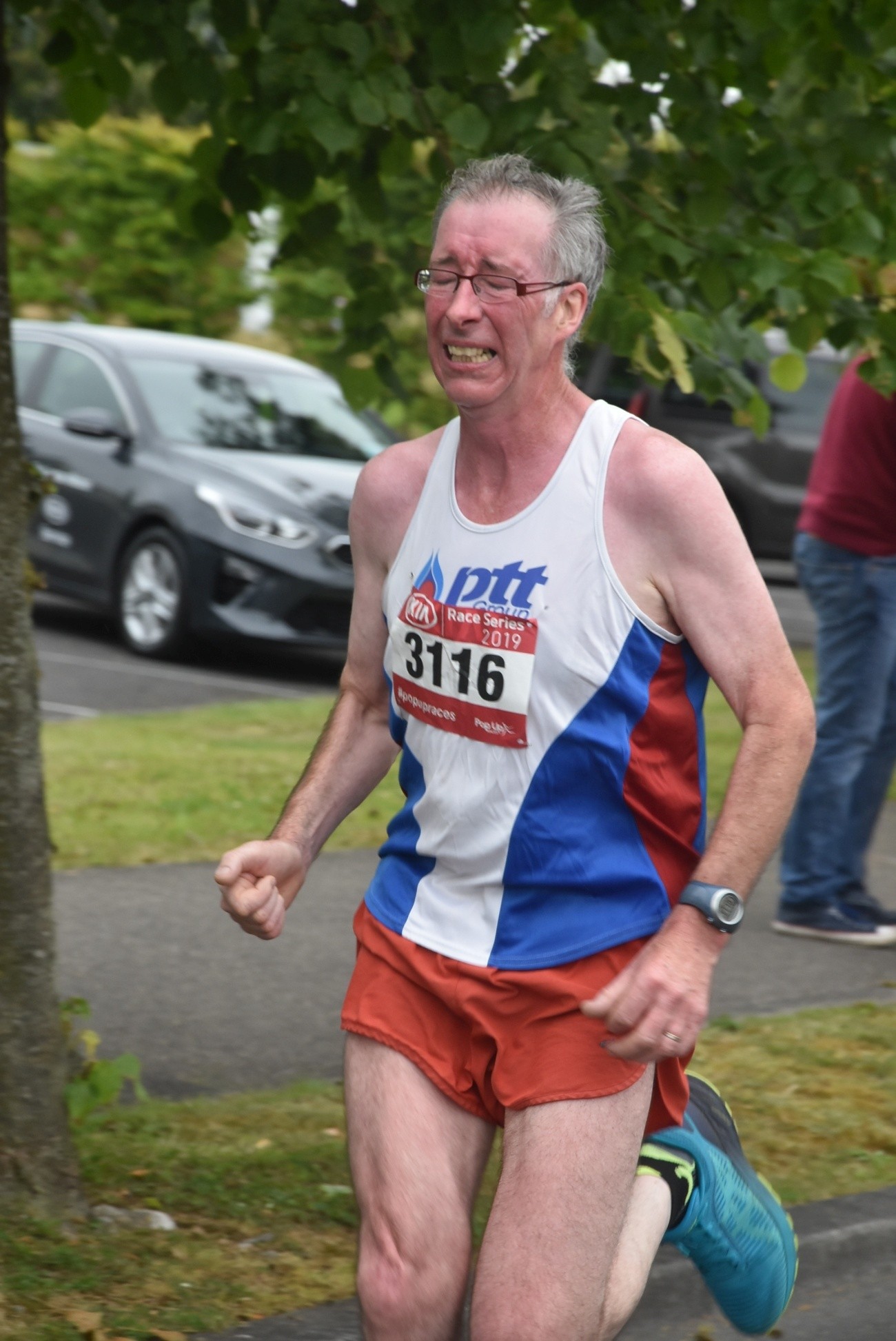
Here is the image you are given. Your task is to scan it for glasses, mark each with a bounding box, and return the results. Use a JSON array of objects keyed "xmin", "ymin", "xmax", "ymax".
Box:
[{"xmin": 414, "ymin": 268, "xmax": 575, "ymax": 305}]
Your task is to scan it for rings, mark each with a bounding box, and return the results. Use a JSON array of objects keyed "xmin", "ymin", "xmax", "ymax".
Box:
[{"xmin": 662, "ymin": 1031, "xmax": 682, "ymax": 1043}]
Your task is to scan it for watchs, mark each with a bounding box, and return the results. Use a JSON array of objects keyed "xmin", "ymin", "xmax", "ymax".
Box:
[{"xmin": 678, "ymin": 880, "xmax": 745, "ymax": 934}]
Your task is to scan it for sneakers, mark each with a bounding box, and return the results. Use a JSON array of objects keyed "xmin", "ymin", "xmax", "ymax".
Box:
[
  {"xmin": 772, "ymin": 902, "xmax": 896, "ymax": 945},
  {"xmin": 841, "ymin": 891, "xmax": 896, "ymax": 923},
  {"xmin": 645, "ymin": 1068, "xmax": 799, "ymax": 1335}
]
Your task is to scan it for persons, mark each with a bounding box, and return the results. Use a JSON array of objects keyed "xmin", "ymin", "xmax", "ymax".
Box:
[
  {"xmin": 770, "ymin": 307, "xmax": 896, "ymax": 948},
  {"xmin": 214, "ymin": 155, "xmax": 817, "ymax": 1341}
]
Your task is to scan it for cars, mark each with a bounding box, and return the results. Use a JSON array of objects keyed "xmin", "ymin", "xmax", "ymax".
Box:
[
  {"xmin": 4, "ymin": 315, "xmax": 396, "ymax": 680},
  {"xmin": 568, "ymin": 306, "xmax": 857, "ymax": 569}
]
